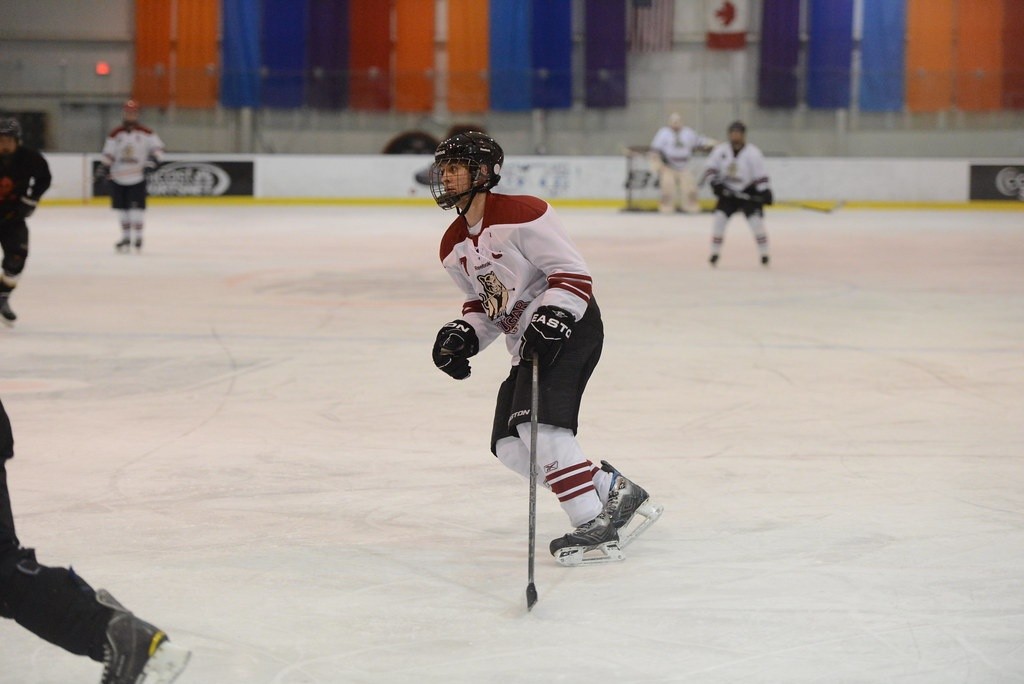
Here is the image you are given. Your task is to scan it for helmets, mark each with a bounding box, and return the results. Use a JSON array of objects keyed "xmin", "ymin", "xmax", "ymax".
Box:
[
  {"xmin": 729, "ymin": 122, "xmax": 745, "ymax": 151},
  {"xmin": 121, "ymin": 100, "xmax": 139, "ymax": 133},
  {"xmin": 0, "ymin": 117, "xmax": 22, "ymax": 146},
  {"xmin": 429, "ymin": 132, "xmax": 504, "ymax": 210}
]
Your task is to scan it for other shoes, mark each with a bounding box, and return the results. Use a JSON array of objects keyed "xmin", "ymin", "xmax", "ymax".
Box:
[
  {"xmin": 761, "ymin": 256, "xmax": 768, "ymax": 263},
  {"xmin": 135, "ymin": 239, "xmax": 142, "ymax": 246},
  {"xmin": 116, "ymin": 238, "xmax": 130, "ymax": 246},
  {"xmin": 710, "ymin": 256, "xmax": 718, "ymax": 262}
]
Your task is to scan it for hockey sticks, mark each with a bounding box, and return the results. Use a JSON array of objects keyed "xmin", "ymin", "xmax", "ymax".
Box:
[
  {"xmin": 734, "ymin": 193, "xmax": 848, "ymax": 213},
  {"xmin": 524, "ymin": 351, "xmax": 541, "ymax": 612},
  {"xmin": 107, "ymin": 160, "xmax": 175, "ymax": 180}
]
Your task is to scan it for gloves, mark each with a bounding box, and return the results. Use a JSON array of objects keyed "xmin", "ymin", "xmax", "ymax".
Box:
[
  {"xmin": 143, "ymin": 156, "xmax": 158, "ymax": 174},
  {"xmin": 752, "ymin": 189, "xmax": 772, "ymax": 208},
  {"xmin": 13, "ymin": 196, "xmax": 36, "ymax": 222},
  {"xmin": 710, "ymin": 179, "xmax": 726, "ymax": 197},
  {"xmin": 519, "ymin": 305, "xmax": 577, "ymax": 374},
  {"xmin": 93, "ymin": 165, "xmax": 109, "ymax": 183},
  {"xmin": 433, "ymin": 320, "xmax": 479, "ymax": 380}
]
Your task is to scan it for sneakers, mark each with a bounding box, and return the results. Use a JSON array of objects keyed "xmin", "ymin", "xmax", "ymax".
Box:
[
  {"xmin": 549, "ymin": 486, "xmax": 626, "ymax": 567},
  {"xmin": 601, "ymin": 460, "xmax": 664, "ymax": 548},
  {"xmin": 95, "ymin": 589, "xmax": 192, "ymax": 684},
  {"xmin": 0, "ymin": 278, "xmax": 17, "ymax": 326}
]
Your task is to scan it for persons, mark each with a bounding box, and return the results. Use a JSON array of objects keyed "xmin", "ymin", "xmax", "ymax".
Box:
[
  {"xmin": 428, "ymin": 131, "xmax": 663, "ymax": 566},
  {"xmin": 706, "ymin": 121, "xmax": 774, "ymax": 269},
  {"xmin": 96, "ymin": 100, "xmax": 164, "ymax": 251},
  {"xmin": 652, "ymin": 116, "xmax": 700, "ymax": 213},
  {"xmin": 0, "ymin": 118, "xmax": 51, "ymax": 327},
  {"xmin": 0, "ymin": 399, "xmax": 190, "ymax": 684}
]
